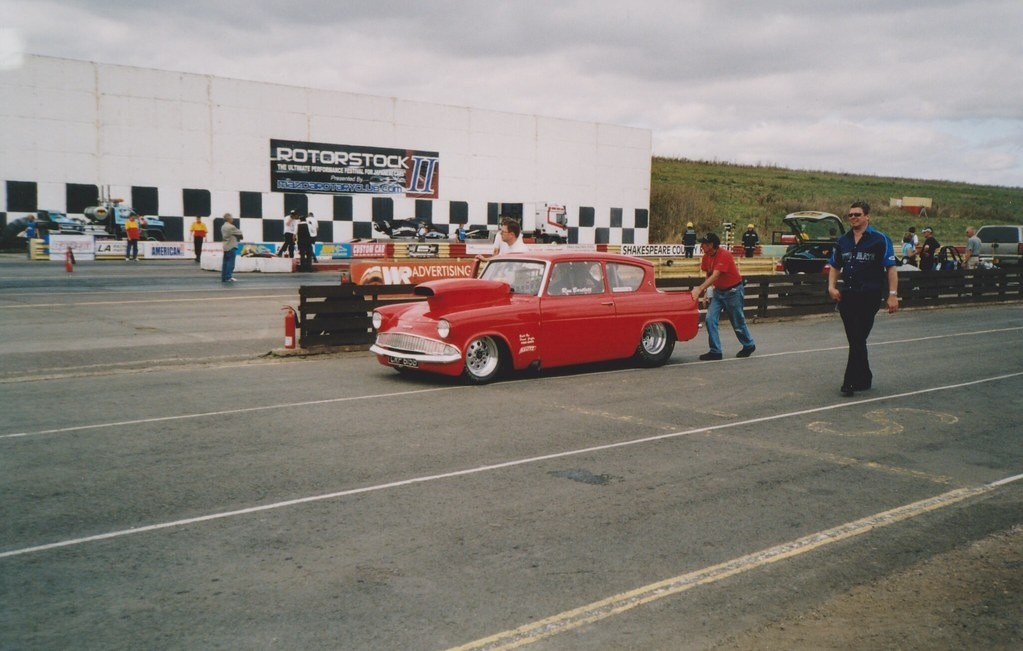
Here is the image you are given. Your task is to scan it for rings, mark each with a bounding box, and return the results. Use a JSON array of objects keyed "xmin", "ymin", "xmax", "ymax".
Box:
[{"xmin": 889, "ymin": 291, "xmax": 897, "ymax": 296}]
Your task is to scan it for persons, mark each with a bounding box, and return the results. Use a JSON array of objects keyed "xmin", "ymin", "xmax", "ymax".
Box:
[
  {"xmin": 691, "ymin": 233, "xmax": 756, "ymax": 361},
  {"xmin": 476, "ymin": 217, "xmax": 527, "ymax": 286},
  {"xmin": 220, "ymin": 214, "xmax": 243, "ymax": 283},
  {"xmin": 277, "ymin": 208, "xmax": 319, "ymax": 263},
  {"xmin": 786, "ymin": 232, "xmax": 809, "ymax": 286},
  {"xmin": 816, "ymin": 228, "xmax": 839, "ymax": 274},
  {"xmin": 901, "ymin": 227, "xmax": 920, "ymax": 267},
  {"xmin": 455, "ymin": 223, "xmax": 466, "ymax": 243},
  {"xmin": 19, "ymin": 215, "xmax": 37, "ymax": 258},
  {"xmin": 190, "ymin": 216, "xmax": 208, "ymax": 263},
  {"xmin": 960, "ymin": 226, "xmax": 982, "ymax": 296},
  {"xmin": 682, "ymin": 222, "xmax": 696, "ymax": 258},
  {"xmin": 416, "ymin": 221, "xmax": 426, "ymax": 241},
  {"xmin": 741, "ymin": 224, "xmax": 759, "ymax": 257},
  {"xmin": 125, "ymin": 212, "xmax": 140, "ymax": 262},
  {"xmin": 919, "ymin": 226, "xmax": 940, "ymax": 300},
  {"xmin": 828, "ymin": 202, "xmax": 899, "ymax": 394}
]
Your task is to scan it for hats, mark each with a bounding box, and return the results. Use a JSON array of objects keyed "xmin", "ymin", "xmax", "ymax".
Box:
[
  {"xmin": 697, "ymin": 233, "xmax": 721, "ymax": 245},
  {"xmin": 922, "ymin": 227, "xmax": 933, "ymax": 233}
]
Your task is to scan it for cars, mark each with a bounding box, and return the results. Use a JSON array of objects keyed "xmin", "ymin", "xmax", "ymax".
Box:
[
  {"xmin": 772, "ymin": 210, "xmax": 967, "ymax": 308},
  {"xmin": 369, "ymin": 249, "xmax": 705, "ymax": 387}
]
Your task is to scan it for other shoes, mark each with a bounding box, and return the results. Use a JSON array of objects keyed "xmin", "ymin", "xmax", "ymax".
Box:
[
  {"xmin": 126, "ymin": 258, "xmax": 130, "ymax": 262},
  {"xmin": 133, "ymin": 259, "xmax": 140, "ymax": 261},
  {"xmin": 700, "ymin": 352, "xmax": 722, "ymax": 360},
  {"xmin": 736, "ymin": 344, "xmax": 756, "ymax": 358},
  {"xmin": 855, "ymin": 380, "xmax": 872, "ymax": 390},
  {"xmin": 223, "ymin": 278, "xmax": 237, "ymax": 282},
  {"xmin": 840, "ymin": 383, "xmax": 853, "ymax": 395}
]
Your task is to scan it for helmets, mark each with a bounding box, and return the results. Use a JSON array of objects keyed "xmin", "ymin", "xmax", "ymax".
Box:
[
  {"xmin": 747, "ymin": 224, "xmax": 755, "ymax": 228},
  {"xmin": 686, "ymin": 222, "xmax": 694, "ymax": 227}
]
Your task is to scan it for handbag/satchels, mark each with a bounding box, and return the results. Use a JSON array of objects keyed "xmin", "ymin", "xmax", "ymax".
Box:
[{"xmin": 902, "ymin": 256, "xmax": 918, "ymax": 267}]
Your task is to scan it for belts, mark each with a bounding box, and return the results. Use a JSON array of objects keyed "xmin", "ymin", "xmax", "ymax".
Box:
[
  {"xmin": 970, "ymin": 255, "xmax": 979, "ymax": 257},
  {"xmin": 718, "ymin": 280, "xmax": 742, "ymax": 293}
]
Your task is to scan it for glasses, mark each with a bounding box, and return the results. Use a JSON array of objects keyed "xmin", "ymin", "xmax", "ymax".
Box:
[
  {"xmin": 501, "ymin": 230, "xmax": 511, "ymax": 234},
  {"xmin": 847, "ymin": 212, "xmax": 866, "ymax": 217}
]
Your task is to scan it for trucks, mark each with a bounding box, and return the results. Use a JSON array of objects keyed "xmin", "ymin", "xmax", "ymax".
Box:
[{"xmin": 0, "ymin": 197, "xmax": 167, "ymax": 251}]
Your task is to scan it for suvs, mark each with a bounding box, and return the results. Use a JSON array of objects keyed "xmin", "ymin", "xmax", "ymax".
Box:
[{"xmin": 974, "ymin": 224, "xmax": 1023, "ymax": 274}]
